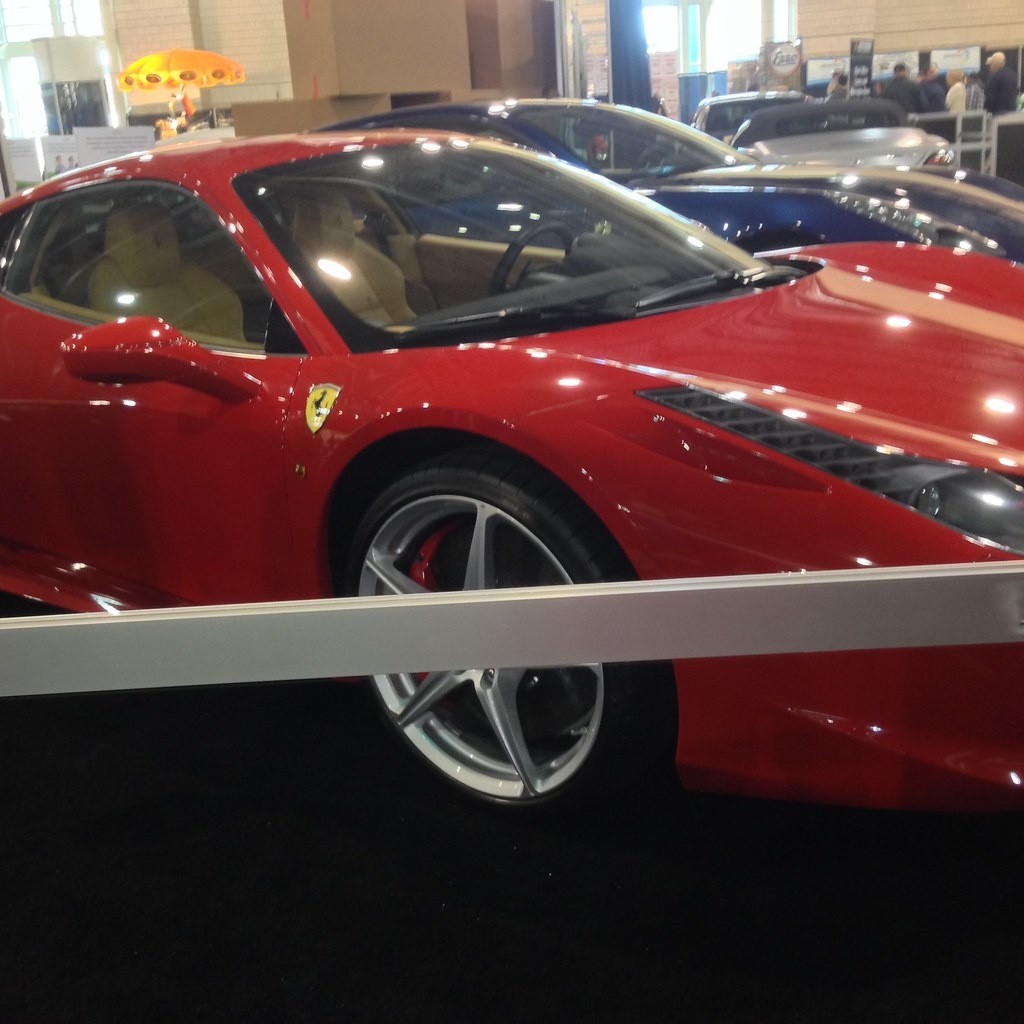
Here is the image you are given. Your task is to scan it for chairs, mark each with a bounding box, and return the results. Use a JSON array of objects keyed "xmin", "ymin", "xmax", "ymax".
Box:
[
  {"xmin": 290, "ymin": 186, "xmax": 417, "ymax": 327},
  {"xmin": 87, "ymin": 202, "xmax": 246, "ymax": 344}
]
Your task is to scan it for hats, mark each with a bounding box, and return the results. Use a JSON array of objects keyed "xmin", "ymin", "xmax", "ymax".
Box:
[
  {"xmin": 986, "ymin": 52, "xmax": 1004, "ymax": 65},
  {"xmin": 949, "ymin": 69, "xmax": 964, "ymax": 80}
]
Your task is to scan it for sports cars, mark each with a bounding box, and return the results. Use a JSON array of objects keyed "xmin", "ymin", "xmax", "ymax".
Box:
[{"xmin": 0, "ymin": 127, "xmax": 1024, "ymax": 826}]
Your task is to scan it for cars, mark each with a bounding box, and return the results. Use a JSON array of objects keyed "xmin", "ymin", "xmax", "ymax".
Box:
[{"xmin": 272, "ymin": 90, "xmax": 1024, "ymax": 261}]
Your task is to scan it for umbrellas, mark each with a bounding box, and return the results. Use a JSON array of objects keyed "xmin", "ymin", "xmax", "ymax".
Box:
[{"xmin": 119, "ymin": 49, "xmax": 247, "ymax": 90}]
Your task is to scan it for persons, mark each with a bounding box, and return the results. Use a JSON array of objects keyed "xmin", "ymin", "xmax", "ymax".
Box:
[{"xmin": 827, "ymin": 51, "xmax": 1017, "ymax": 114}]
